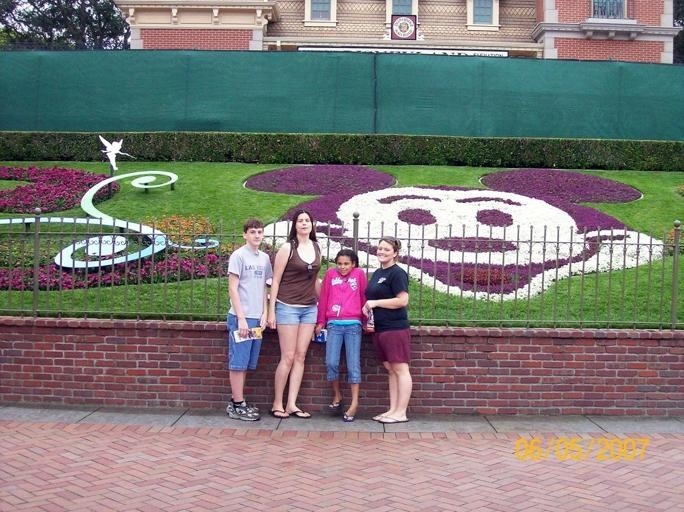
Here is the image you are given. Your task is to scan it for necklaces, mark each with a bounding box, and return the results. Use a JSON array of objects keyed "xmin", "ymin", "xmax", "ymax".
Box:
[
  {"xmin": 299, "ymin": 245, "xmax": 315, "ymax": 273},
  {"xmin": 381, "ymin": 263, "xmax": 395, "ymax": 270}
]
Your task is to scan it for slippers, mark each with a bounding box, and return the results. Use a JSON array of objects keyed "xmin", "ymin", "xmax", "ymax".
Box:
[
  {"xmin": 372, "ymin": 411, "xmax": 409, "ymax": 423},
  {"xmin": 343, "ymin": 411, "xmax": 356, "ymax": 422},
  {"xmin": 328, "ymin": 401, "xmax": 343, "ymax": 410},
  {"xmin": 269, "ymin": 408, "xmax": 290, "ymax": 418},
  {"xmin": 290, "ymin": 409, "xmax": 311, "ymax": 418}
]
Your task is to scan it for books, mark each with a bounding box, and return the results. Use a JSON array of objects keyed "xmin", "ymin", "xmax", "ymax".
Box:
[
  {"xmin": 311, "ymin": 328, "xmax": 327, "ymax": 343},
  {"xmin": 232, "ymin": 327, "xmax": 264, "ymax": 345}
]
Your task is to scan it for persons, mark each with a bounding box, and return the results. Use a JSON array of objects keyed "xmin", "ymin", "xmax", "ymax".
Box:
[
  {"xmin": 361, "ymin": 234, "xmax": 412, "ymax": 423},
  {"xmin": 314, "ymin": 249, "xmax": 370, "ymax": 422},
  {"xmin": 223, "ymin": 220, "xmax": 272, "ymax": 423},
  {"xmin": 267, "ymin": 208, "xmax": 323, "ymax": 420}
]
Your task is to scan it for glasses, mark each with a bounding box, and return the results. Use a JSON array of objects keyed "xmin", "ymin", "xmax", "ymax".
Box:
[{"xmin": 308, "ymin": 265, "xmax": 313, "ymax": 279}]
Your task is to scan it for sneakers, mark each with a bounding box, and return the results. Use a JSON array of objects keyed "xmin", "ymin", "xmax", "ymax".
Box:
[{"xmin": 226, "ymin": 401, "xmax": 260, "ymax": 420}]
